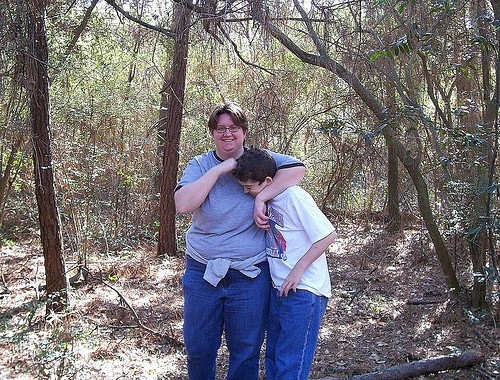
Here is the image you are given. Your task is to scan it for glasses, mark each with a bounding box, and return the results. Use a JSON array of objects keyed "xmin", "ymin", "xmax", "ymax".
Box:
[{"xmin": 214, "ymin": 123, "xmax": 241, "ymax": 133}]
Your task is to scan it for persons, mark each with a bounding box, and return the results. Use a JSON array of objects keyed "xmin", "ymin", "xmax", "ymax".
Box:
[
  {"xmin": 173, "ymin": 103, "xmax": 305, "ymax": 380},
  {"xmin": 234, "ymin": 147, "xmax": 338, "ymax": 380}
]
